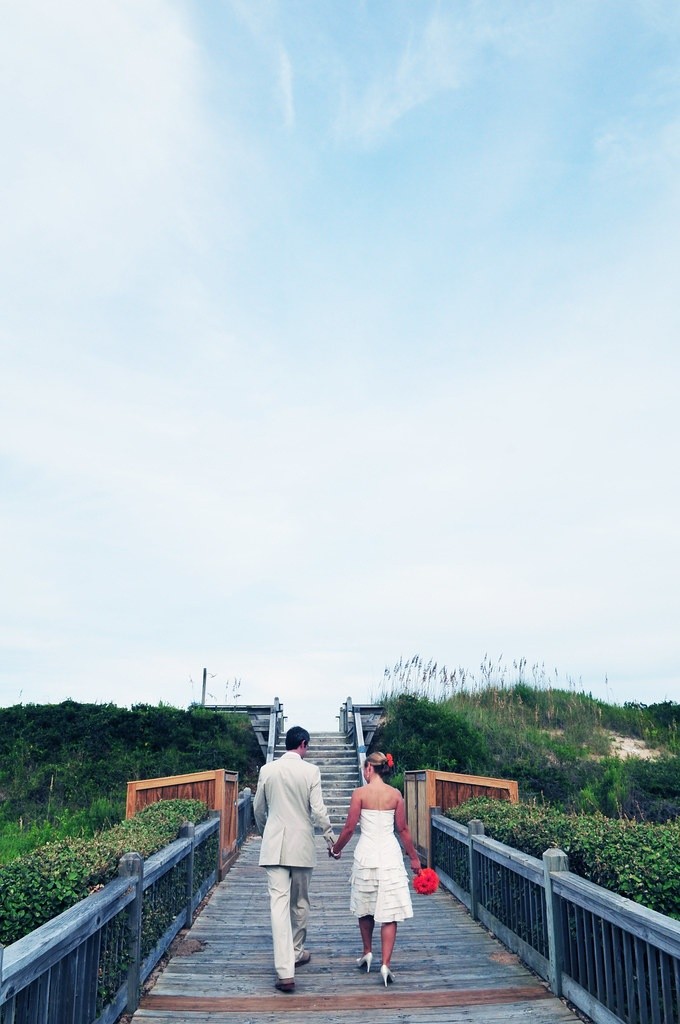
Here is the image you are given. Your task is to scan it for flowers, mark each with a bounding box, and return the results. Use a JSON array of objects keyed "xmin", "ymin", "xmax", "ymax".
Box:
[
  {"xmin": 385, "ymin": 753, "xmax": 394, "ymax": 767},
  {"xmin": 412, "ymin": 867, "xmax": 440, "ymax": 896}
]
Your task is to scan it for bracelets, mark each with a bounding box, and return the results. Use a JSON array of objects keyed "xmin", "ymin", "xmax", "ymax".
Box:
[{"xmin": 331, "ymin": 846, "xmax": 340, "ymax": 856}]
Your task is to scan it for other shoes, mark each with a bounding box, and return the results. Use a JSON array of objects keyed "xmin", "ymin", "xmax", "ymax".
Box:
[
  {"xmin": 295, "ymin": 951, "xmax": 310, "ymax": 968},
  {"xmin": 275, "ymin": 978, "xmax": 295, "ymax": 992}
]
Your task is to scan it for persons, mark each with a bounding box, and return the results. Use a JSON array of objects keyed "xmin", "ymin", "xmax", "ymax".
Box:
[
  {"xmin": 331, "ymin": 752, "xmax": 421, "ymax": 987},
  {"xmin": 252, "ymin": 727, "xmax": 341, "ymax": 989}
]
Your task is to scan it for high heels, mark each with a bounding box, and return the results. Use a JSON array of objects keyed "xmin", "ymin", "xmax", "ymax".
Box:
[
  {"xmin": 380, "ymin": 965, "xmax": 395, "ymax": 987},
  {"xmin": 356, "ymin": 952, "xmax": 372, "ymax": 972}
]
